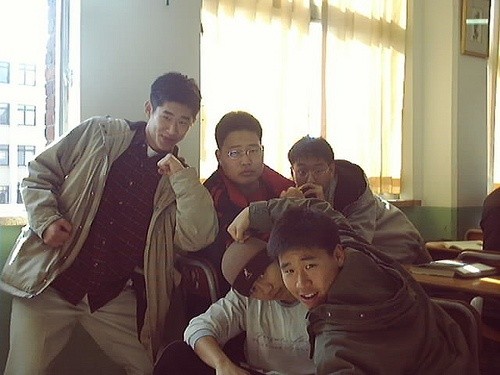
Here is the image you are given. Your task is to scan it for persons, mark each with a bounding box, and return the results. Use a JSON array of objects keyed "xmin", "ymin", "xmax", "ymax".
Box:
[
  {"xmin": 0, "ymin": 71, "xmax": 220, "ymax": 375},
  {"xmin": 150, "ymin": 109, "xmax": 500, "ymax": 375}
]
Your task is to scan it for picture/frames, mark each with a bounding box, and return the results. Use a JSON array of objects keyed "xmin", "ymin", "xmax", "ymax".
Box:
[{"xmin": 461, "ymin": 0, "xmax": 491, "ymax": 57}]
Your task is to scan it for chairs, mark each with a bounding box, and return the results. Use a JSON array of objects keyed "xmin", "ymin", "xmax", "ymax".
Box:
[
  {"xmin": 458, "ymin": 229, "xmax": 500, "ymax": 270},
  {"xmin": 430, "ymin": 298, "xmax": 483, "ymax": 375},
  {"xmin": 175, "ymin": 254, "xmax": 219, "ymax": 320}
]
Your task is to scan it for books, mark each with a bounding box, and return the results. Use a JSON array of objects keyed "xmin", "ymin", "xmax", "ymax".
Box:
[
  {"xmin": 423, "ymin": 258, "xmax": 497, "ymax": 278},
  {"xmin": 425, "ymin": 240, "xmax": 484, "ymax": 251}
]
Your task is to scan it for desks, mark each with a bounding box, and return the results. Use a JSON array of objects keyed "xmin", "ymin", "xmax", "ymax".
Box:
[{"xmin": 413, "ymin": 241, "xmax": 500, "ymax": 343}]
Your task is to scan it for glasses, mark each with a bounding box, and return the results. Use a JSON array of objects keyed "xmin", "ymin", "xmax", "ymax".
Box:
[
  {"xmin": 219, "ymin": 145, "xmax": 267, "ymax": 160},
  {"xmin": 292, "ymin": 167, "xmax": 331, "ymax": 177}
]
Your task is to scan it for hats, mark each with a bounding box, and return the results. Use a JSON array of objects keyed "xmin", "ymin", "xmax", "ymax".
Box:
[{"xmin": 221, "ymin": 237, "xmax": 275, "ymax": 295}]
